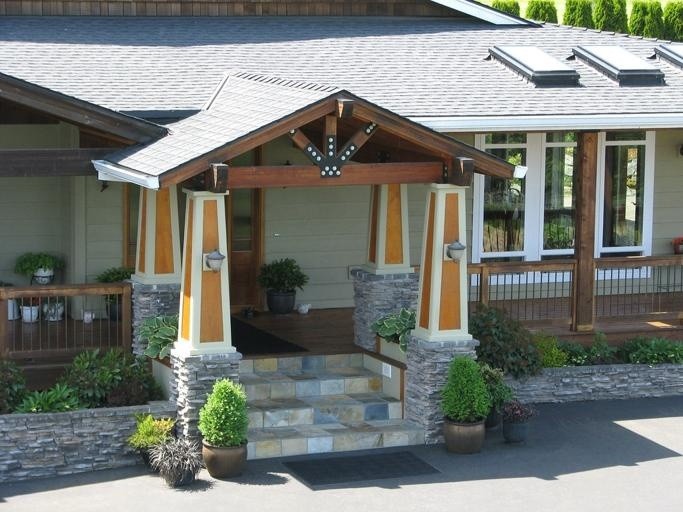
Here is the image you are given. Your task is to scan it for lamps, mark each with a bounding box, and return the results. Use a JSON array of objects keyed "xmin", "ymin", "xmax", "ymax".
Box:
[
  {"xmin": 444, "ymin": 240, "xmax": 465, "ymax": 265},
  {"xmin": 202, "ymin": 250, "xmax": 225, "ymax": 275}
]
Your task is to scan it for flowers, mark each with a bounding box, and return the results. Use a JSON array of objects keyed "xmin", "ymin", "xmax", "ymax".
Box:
[
  {"xmin": 126, "ymin": 411, "xmax": 178, "ymax": 450},
  {"xmin": 671, "ymin": 235, "xmax": 682, "ymax": 245},
  {"xmin": 499, "ymin": 400, "xmax": 539, "ymax": 424}
]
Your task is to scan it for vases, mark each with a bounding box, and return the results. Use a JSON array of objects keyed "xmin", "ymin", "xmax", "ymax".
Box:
[
  {"xmin": 503, "ymin": 423, "xmax": 527, "ymax": 443},
  {"xmin": 673, "ymin": 245, "xmax": 682, "ymax": 254},
  {"xmin": 139, "ymin": 446, "xmax": 165, "ymax": 473}
]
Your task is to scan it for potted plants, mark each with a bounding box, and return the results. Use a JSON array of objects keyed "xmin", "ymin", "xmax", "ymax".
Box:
[
  {"xmin": 150, "ymin": 437, "xmax": 206, "ymax": 489},
  {"xmin": 256, "ymin": 257, "xmax": 310, "ymax": 314},
  {"xmin": 197, "ymin": 375, "xmax": 250, "ymax": 489},
  {"xmin": 438, "ymin": 354, "xmax": 515, "ymax": 455},
  {"xmin": 97, "ymin": 268, "xmax": 135, "ymax": 321},
  {"xmin": 2, "ymin": 252, "xmax": 64, "ymax": 323}
]
